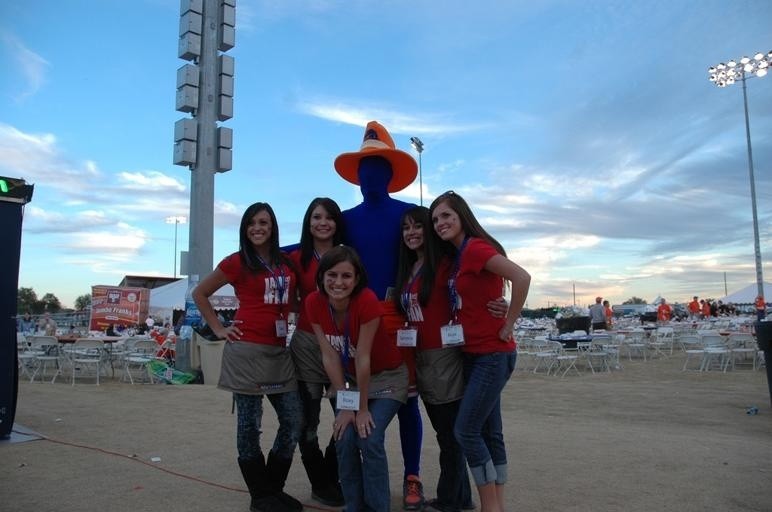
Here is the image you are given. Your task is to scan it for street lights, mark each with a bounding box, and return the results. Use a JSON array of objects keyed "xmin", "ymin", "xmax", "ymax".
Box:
[
  {"xmin": 165, "ymin": 215, "xmax": 187, "ymax": 276},
  {"xmin": 409, "ymin": 136, "xmax": 425, "ymax": 207},
  {"xmin": 708, "ymin": 51, "xmax": 770, "ymax": 296}
]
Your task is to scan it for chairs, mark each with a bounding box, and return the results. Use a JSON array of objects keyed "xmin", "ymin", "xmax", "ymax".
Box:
[
  {"xmin": 514, "ymin": 315, "xmax": 766, "ymax": 378},
  {"xmin": 17, "ymin": 329, "xmax": 176, "ymax": 385}
]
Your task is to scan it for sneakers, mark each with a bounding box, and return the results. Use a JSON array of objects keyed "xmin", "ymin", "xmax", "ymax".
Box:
[{"xmin": 402, "ymin": 473, "xmax": 427, "ymax": 511}]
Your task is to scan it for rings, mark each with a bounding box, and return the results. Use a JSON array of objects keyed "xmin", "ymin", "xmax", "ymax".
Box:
[
  {"xmin": 334, "ymin": 424, "xmax": 337, "ymax": 427},
  {"xmin": 361, "ymin": 425, "xmax": 365, "ymax": 426}
]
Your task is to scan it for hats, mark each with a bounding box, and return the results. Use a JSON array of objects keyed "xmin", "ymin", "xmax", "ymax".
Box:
[
  {"xmin": 596, "ymin": 297, "xmax": 602, "ymax": 302},
  {"xmin": 334, "ymin": 120, "xmax": 419, "ymax": 194},
  {"xmin": 693, "ymin": 296, "xmax": 698, "ymax": 299}
]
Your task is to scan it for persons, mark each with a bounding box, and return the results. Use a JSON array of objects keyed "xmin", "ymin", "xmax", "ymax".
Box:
[
  {"xmin": 192, "ymin": 203, "xmax": 307, "ymax": 512},
  {"xmin": 217, "ymin": 312, "xmax": 225, "ymax": 322},
  {"xmin": 394, "ymin": 206, "xmax": 508, "ymax": 512},
  {"xmin": 657, "ymin": 298, "xmax": 671, "ymax": 321},
  {"xmin": 701, "ymin": 299, "xmax": 736, "ymax": 318},
  {"xmin": 145, "ymin": 314, "xmax": 176, "ymax": 367},
  {"xmin": 687, "ymin": 296, "xmax": 701, "ymax": 319},
  {"xmin": 589, "ymin": 297, "xmax": 607, "ymax": 332},
  {"xmin": 37, "ymin": 313, "xmax": 58, "ymax": 355},
  {"xmin": 276, "ymin": 121, "xmax": 434, "ymax": 510},
  {"xmin": 428, "ymin": 190, "xmax": 531, "ymax": 512},
  {"xmin": 287, "ymin": 197, "xmax": 345, "ymax": 506},
  {"xmin": 19, "ymin": 311, "xmax": 36, "ymax": 347},
  {"xmin": 105, "ymin": 320, "xmax": 127, "ymax": 337},
  {"xmin": 754, "ymin": 296, "xmax": 767, "ymax": 320},
  {"xmin": 304, "ymin": 245, "xmax": 410, "ymax": 512},
  {"xmin": 603, "ymin": 300, "xmax": 612, "ymax": 326}
]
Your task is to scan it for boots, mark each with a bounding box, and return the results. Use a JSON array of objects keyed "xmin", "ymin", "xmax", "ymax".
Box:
[
  {"xmin": 251, "ymin": 447, "xmax": 293, "ymax": 512},
  {"xmin": 237, "ymin": 449, "xmax": 302, "ymax": 512},
  {"xmin": 299, "ymin": 445, "xmax": 346, "ymax": 507},
  {"xmin": 427, "ymin": 450, "xmax": 477, "ymax": 511}
]
